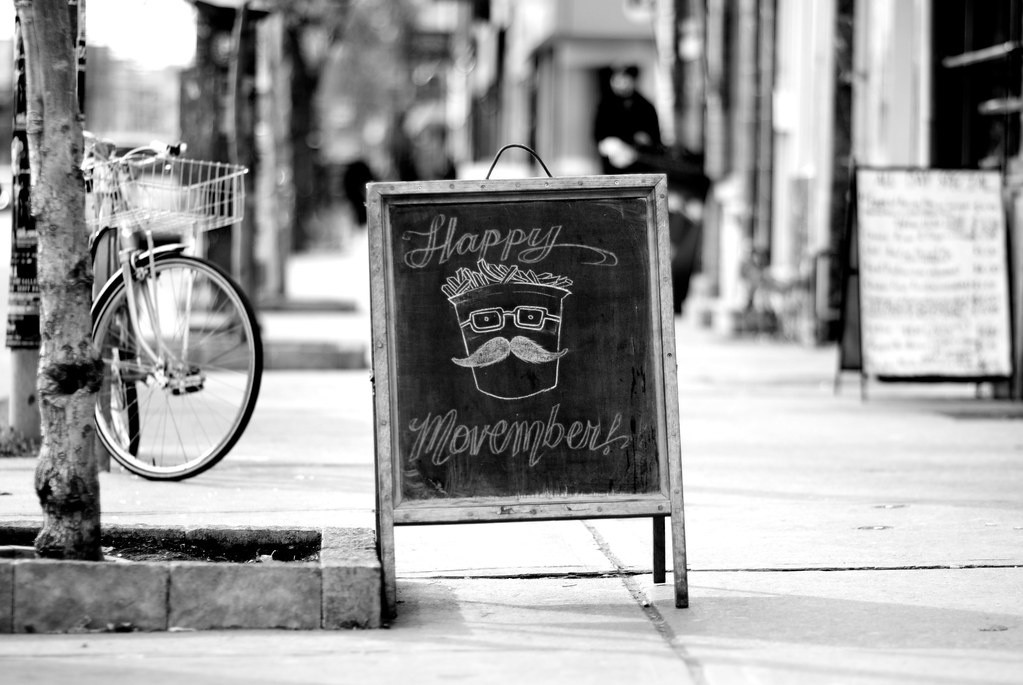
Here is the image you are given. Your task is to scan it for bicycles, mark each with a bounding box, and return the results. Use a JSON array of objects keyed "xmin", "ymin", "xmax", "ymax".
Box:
[{"xmin": 83, "ymin": 128, "xmax": 263, "ymax": 483}]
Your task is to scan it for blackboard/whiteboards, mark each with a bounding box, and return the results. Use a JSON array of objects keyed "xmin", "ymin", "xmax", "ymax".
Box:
[
  {"xmin": 361, "ymin": 172, "xmax": 685, "ymax": 523},
  {"xmin": 837, "ymin": 161, "xmax": 1016, "ymax": 383}
]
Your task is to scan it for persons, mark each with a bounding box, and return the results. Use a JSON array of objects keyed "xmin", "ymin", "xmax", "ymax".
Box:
[
  {"xmin": 343, "ymin": 159, "xmax": 376, "ymax": 225},
  {"xmin": 401, "ymin": 109, "xmax": 457, "ymax": 181},
  {"xmin": 591, "ymin": 63, "xmax": 661, "ymax": 176}
]
